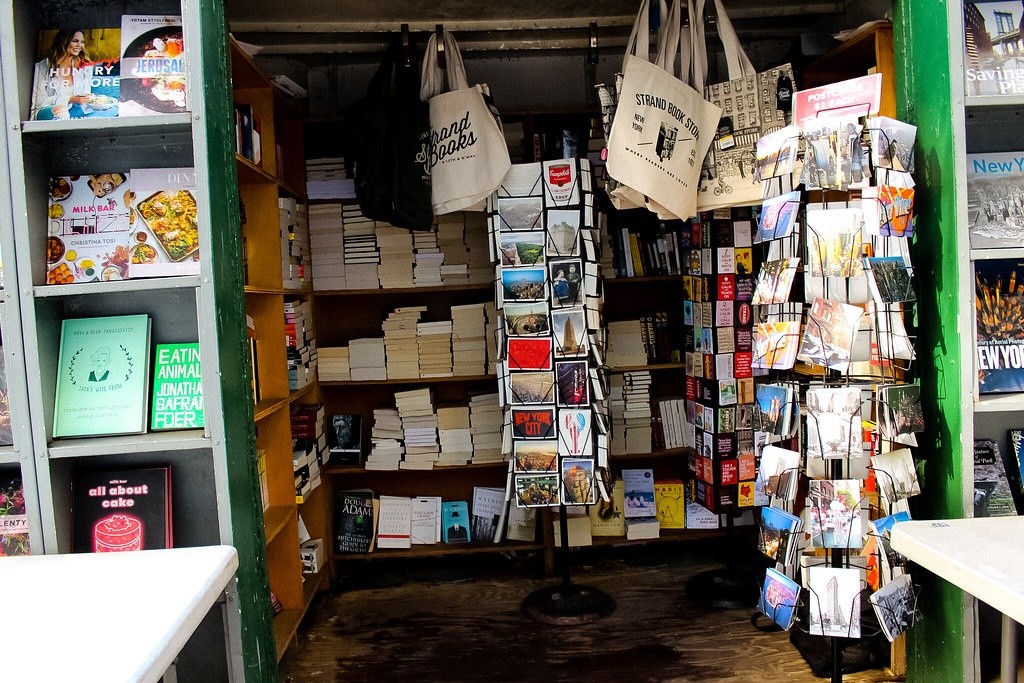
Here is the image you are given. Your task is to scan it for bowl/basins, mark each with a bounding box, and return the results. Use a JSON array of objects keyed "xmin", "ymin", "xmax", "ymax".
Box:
[
  {"xmin": 65, "ymin": 249, "xmax": 77, "ymax": 262},
  {"xmin": 47, "ymin": 236, "xmax": 66, "ymax": 265},
  {"xmin": 133, "ymin": 231, "xmax": 147, "ymax": 244},
  {"xmin": 48, "ymin": 202, "xmax": 65, "ymax": 220},
  {"xmin": 101, "ymin": 265, "xmax": 121, "ymax": 281},
  {"xmin": 120, "ymin": 25, "xmax": 187, "ymax": 114},
  {"xmin": 48, "ymin": 177, "xmax": 73, "ymax": 202},
  {"xmin": 87, "ymin": 96, "xmax": 118, "ymax": 111}
]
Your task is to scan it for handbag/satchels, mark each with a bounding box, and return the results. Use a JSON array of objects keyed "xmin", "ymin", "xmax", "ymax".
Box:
[
  {"xmin": 348, "ymin": 31, "xmax": 513, "ymax": 234},
  {"xmin": 598, "ymin": 0, "xmax": 799, "ymax": 223}
]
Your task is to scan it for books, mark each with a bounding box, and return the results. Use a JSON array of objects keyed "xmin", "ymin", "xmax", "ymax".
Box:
[
  {"xmin": 52, "ymin": 313, "xmax": 204, "ymax": 438},
  {"xmin": 45, "ymin": 169, "xmax": 202, "ymax": 284},
  {"xmin": 962, "ymin": 0, "xmax": 1023, "ymax": 515},
  {"xmin": 30, "ymin": 14, "xmax": 191, "ymax": 116},
  {"xmin": 71, "ymin": 467, "xmax": 173, "ymax": 552},
  {"xmin": 230, "ymin": 74, "xmax": 940, "ymax": 640}
]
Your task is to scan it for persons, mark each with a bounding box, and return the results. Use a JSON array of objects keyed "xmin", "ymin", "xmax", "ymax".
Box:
[{"xmin": 31, "ymin": 29, "xmax": 95, "ymax": 120}]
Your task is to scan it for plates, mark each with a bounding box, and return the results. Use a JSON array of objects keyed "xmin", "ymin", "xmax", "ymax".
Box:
[
  {"xmin": 128, "ymin": 244, "xmax": 160, "ymax": 264},
  {"xmin": 72, "ymin": 257, "xmax": 99, "ymax": 282},
  {"xmin": 86, "ymin": 173, "xmax": 127, "ymax": 199},
  {"xmin": 129, "ymin": 206, "xmax": 138, "ymax": 234}
]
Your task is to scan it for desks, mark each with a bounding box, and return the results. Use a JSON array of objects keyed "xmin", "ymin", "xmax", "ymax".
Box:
[
  {"xmin": 1, "ymin": 543, "xmax": 239, "ymax": 682},
  {"xmin": 888, "ymin": 517, "xmax": 1024, "ymax": 683}
]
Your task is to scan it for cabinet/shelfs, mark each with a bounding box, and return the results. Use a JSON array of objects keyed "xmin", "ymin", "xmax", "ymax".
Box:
[
  {"xmin": 224, "ymin": 18, "xmax": 894, "ymax": 671},
  {"xmin": 1, "ymin": 1, "xmax": 279, "ymax": 682},
  {"xmin": 891, "ymin": 0, "xmax": 1024, "ymax": 683}
]
